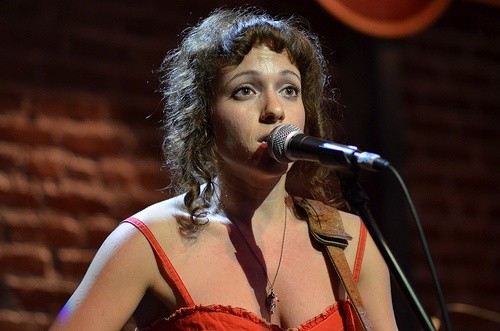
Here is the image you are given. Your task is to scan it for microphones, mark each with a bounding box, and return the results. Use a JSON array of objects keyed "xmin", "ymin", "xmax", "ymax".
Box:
[{"xmin": 267, "ymin": 124, "xmax": 390, "ymax": 173}]
